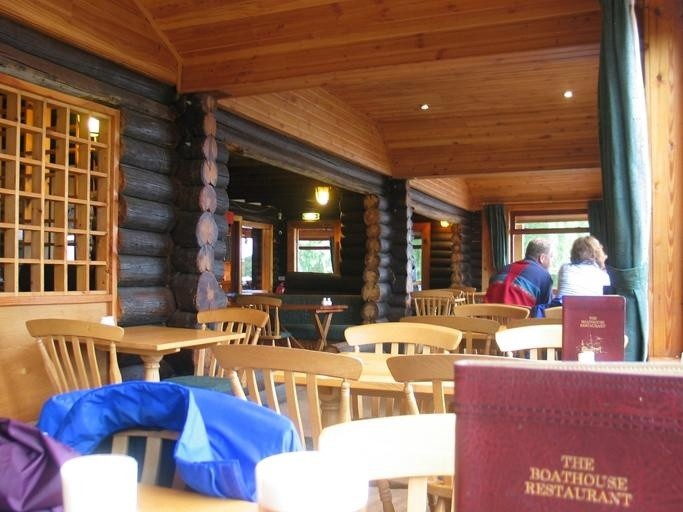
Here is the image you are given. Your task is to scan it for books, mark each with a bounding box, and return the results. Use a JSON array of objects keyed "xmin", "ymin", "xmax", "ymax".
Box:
[
  {"xmin": 562, "ymin": 296, "xmax": 625, "ymax": 361},
  {"xmin": 453, "ymin": 359, "xmax": 683, "ymax": 512}
]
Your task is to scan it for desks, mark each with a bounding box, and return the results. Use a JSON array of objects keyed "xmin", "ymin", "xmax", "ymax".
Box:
[
  {"xmin": 137, "ymin": 480, "xmax": 266, "ymax": 511},
  {"xmin": 94, "ymin": 324, "xmax": 247, "ymax": 382}
]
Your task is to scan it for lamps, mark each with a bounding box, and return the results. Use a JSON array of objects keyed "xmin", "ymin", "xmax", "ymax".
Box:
[{"xmin": 312, "ymin": 181, "xmax": 332, "ymax": 206}]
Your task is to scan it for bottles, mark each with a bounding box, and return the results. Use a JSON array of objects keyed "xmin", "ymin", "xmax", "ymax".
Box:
[{"xmin": 320, "ymin": 297, "xmax": 333, "ymax": 306}]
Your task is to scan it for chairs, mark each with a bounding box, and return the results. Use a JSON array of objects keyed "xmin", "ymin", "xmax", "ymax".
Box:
[
  {"xmin": 342, "ymin": 320, "xmax": 463, "ymax": 420},
  {"xmin": 388, "ymin": 352, "xmax": 538, "ymax": 510},
  {"xmin": 25, "ymin": 317, "xmax": 125, "ymax": 398},
  {"xmin": 216, "ymin": 285, "xmax": 628, "ymax": 408},
  {"xmin": 211, "ymin": 343, "xmax": 364, "ymax": 451},
  {"xmin": 37, "ymin": 378, "xmax": 302, "ymax": 504},
  {"xmin": 316, "ymin": 413, "xmax": 457, "ymax": 512},
  {"xmin": 194, "ymin": 306, "xmax": 271, "ymax": 393}
]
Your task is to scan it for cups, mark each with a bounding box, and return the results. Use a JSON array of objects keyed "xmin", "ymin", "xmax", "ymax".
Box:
[
  {"xmin": 255, "ymin": 451, "xmax": 369, "ymax": 511},
  {"xmin": 57, "ymin": 453, "xmax": 138, "ymax": 511}
]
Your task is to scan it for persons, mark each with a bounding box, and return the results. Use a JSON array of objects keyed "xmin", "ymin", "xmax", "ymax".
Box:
[
  {"xmin": 558, "ymin": 236, "xmax": 611, "ymax": 303},
  {"xmin": 483, "ymin": 240, "xmax": 554, "ymax": 306}
]
